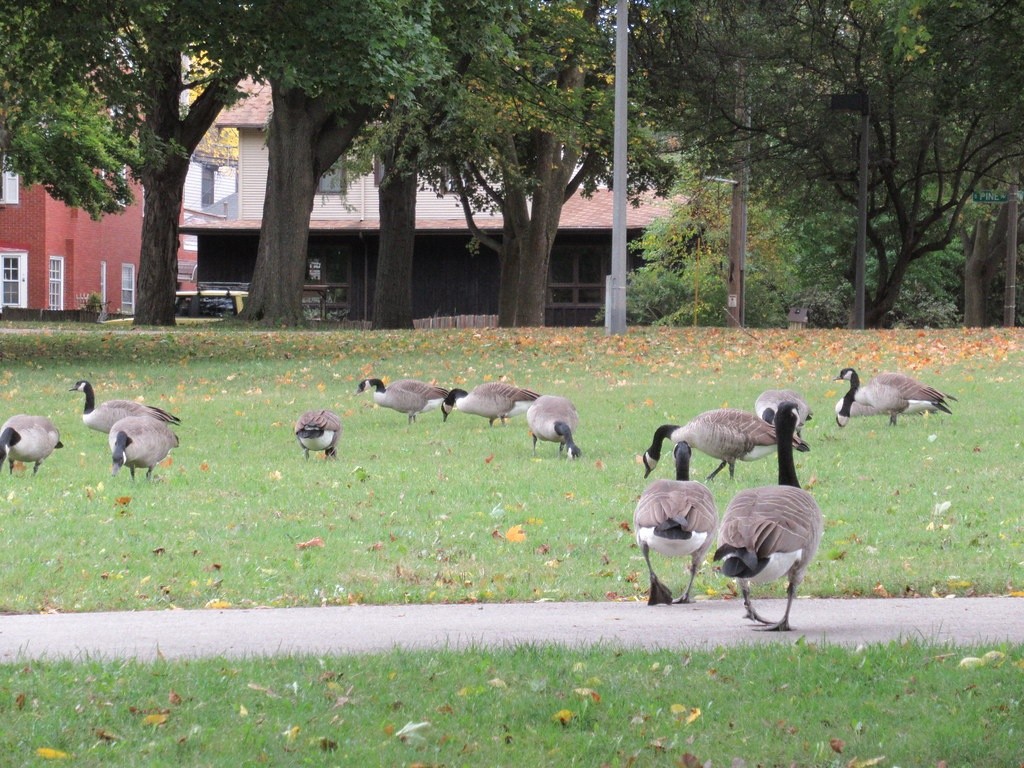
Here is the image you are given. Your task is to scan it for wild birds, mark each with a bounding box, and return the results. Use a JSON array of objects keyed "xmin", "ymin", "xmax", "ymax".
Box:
[
  {"xmin": 294, "ymin": 409, "xmax": 342, "ymax": 459},
  {"xmin": 643, "ymin": 408, "xmax": 810, "ymax": 482},
  {"xmin": 68, "ymin": 380, "xmax": 181, "ymax": 479},
  {"xmin": 526, "ymin": 394, "xmax": 581, "ymax": 460},
  {"xmin": 354, "ymin": 379, "xmax": 452, "ymax": 424},
  {"xmin": 754, "ymin": 389, "xmax": 814, "ymax": 436},
  {"xmin": 1, "ymin": 414, "xmax": 63, "ymax": 475},
  {"xmin": 442, "ymin": 382, "xmax": 542, "ymax": 427},
  {"xmin": 833, "ymin": 368, "xmax": 959, "ymax": 428},
  {"xmin": 715, "ymin": 402, "xmax": 825, "ymax": 632},
  {"xmin": 633, "ymin": 441, "xmax": 719, "ymax": 606}
]
